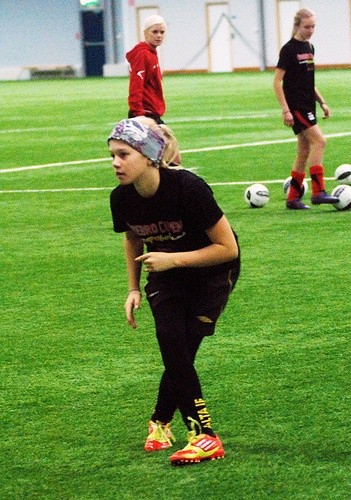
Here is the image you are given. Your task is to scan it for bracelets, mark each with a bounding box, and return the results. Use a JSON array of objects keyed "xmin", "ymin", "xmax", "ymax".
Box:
[
  {"xmin": 128, "ymin": 289, "xmax": 140, "ymax": 292},
  {"xmin": 282, "ymin": 110, "xmax": 291, "ymax": 115}
]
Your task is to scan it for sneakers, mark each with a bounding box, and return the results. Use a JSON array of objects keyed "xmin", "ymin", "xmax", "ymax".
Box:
[
  {"xmin": 311, "ymin": 189, "xmax": 340, "ymax": 205},
  {"xmin": 169, "ymin": 429, "xmax": 225, "ymax": 465},
  {"xmin": 286, "ymin": 197, "xmax": 311, "ymax": 210},
  {"xmin": 144, "ymin": 419, "xmax": 176, "ymax": 453}
]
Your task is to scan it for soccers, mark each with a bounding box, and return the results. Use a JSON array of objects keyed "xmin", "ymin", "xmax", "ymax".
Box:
[
  {"xmin": 283, "ymin": 176, "xmax": 308, "ymax": 199},
  {"xmin": 244, "ymin": 183, "xmax": 269, "ymax": 207},
  {"xmin": 335, "ymin": 164, "xmax": 351, "ymax": 185},
  {"xmin": 331, "ymin": 184, "xmax": 351, "ymax": 211}
]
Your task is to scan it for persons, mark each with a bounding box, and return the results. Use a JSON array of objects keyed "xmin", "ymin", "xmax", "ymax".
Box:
[
  {"xmin": 126, "ymin": 16, "xmax": 166, "ymax": 125},
  {"xmin": 107, "ymin": 116, "xmax": 241, "ymax": 464},
  {"xmin": 273, "ymin": 9, "xmax": 340, "ymax": 210}
]
